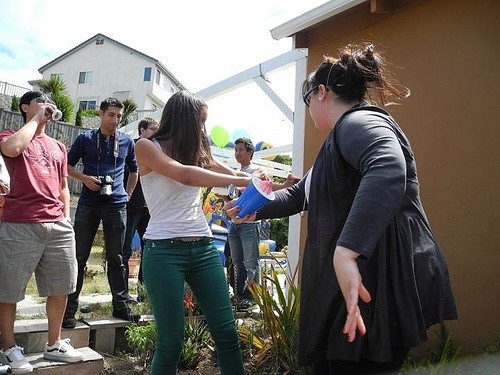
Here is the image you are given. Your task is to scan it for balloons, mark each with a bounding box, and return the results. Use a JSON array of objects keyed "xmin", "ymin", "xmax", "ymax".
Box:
[
  {"xmin": 255, "ymin": 141, "xmax": 276, "ymax": 161},
  {"xmin": 231, "ymin": 128, "xmax": 251, "ymax": 146},
  {"xmin": 211, "ymin": 126, "xmax": 230, "ymax": 147}
]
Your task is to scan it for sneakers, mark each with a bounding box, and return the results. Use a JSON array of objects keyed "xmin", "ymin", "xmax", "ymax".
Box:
[
  {"xmin": 113, "ymin": 308, "xmax": 141, "ymax": 321},
  {"xmin": 0, "ymin": 345, "xmax": 34, "ymax": 374},
  {"xmin": 62, "ymin": 313, "xmax": 78, "ymax": 329},
  {"xmin": 44, "ymin": 338, "xmax": 85, "ymax": 363}
]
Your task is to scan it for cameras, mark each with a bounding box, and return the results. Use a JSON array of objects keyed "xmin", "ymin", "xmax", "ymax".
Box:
[{"xmin": 95, "ymin": 175, "xmax": 114, "ymax": 201}]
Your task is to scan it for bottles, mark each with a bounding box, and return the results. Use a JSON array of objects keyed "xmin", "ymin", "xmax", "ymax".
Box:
[{"xmin": 47, "ymin": 105, "xmax": 62, "ymax": 121}]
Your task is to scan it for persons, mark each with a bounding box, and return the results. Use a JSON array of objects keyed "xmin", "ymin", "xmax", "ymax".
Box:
[
  {"xmin": 62, "ymin": 96, "xmax": 141, "ymax": 329},
  {"xmin": 124, "ymin": 117, "xmax": 160, "ymax": 302},
  {"xmin": 224, "ymin": 45, "xmax": 457, "ymax": 375},
  {"xmin": 223, "ymin": 138, "xmax": 272, "ymax": 311},
  {"xmin": 135, "ymin": 91, "xmax": 299, "ymax": 375},
  {"xmin": 0, "ymin": 91, "xmax": 84, "ymax": 374}
]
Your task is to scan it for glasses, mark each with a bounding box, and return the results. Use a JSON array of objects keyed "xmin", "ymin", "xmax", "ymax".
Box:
[
  {"xmin": 146, "ymin": 126, "xmax": 158, "ymax": 132},
  {"xmin": 35, "ymin": 97, "xmax": 57, "ymax": 105},
  {"xmin": 301, "ymin": 85, "xmax": 330, "ymax": 108}
]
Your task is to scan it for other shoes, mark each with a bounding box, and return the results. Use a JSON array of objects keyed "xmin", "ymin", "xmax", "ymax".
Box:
[{"xmin": 229, "ymin": 295, "xmax": 260, "ymax": 311}]
